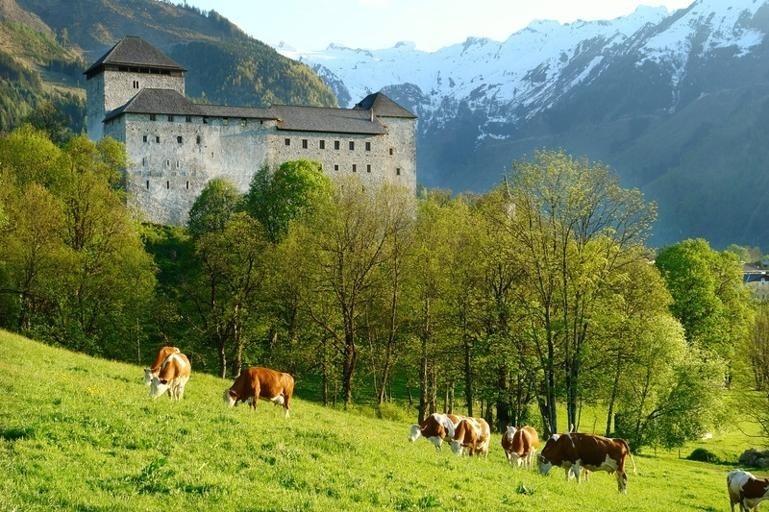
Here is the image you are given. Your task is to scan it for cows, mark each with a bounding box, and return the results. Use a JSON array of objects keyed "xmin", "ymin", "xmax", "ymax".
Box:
[
  {"xmin": 223, "ymin": 366, "xmax": 296, "ymax": 419},
  {"xmin": 499, "ymin": 425, "xmax": 518, "ymax": 464},
  {"xmin": 535, "ymin": 431, "xmax": 638, "ymax": 494},
  {"xmin": 407, "ymin": 412, "xmax": 466, "ymax": 454},
  {"xmin": 143, "ymin": 346, "xmax": 182, "ymax": 388},
  {"xmin": 447, "ymin": 416, "xmax": 491, "ymax": 457},
  {"xmin": 725, "ymin": 467, "xmax": 768, "ymax": 512},
  {"xmin": 508, "ymin": 424, "xmax": 539, "ymax": 472},
  {"xmin": 149, "ymin": 352, "xmax": 191, "ymax": 402}
]
